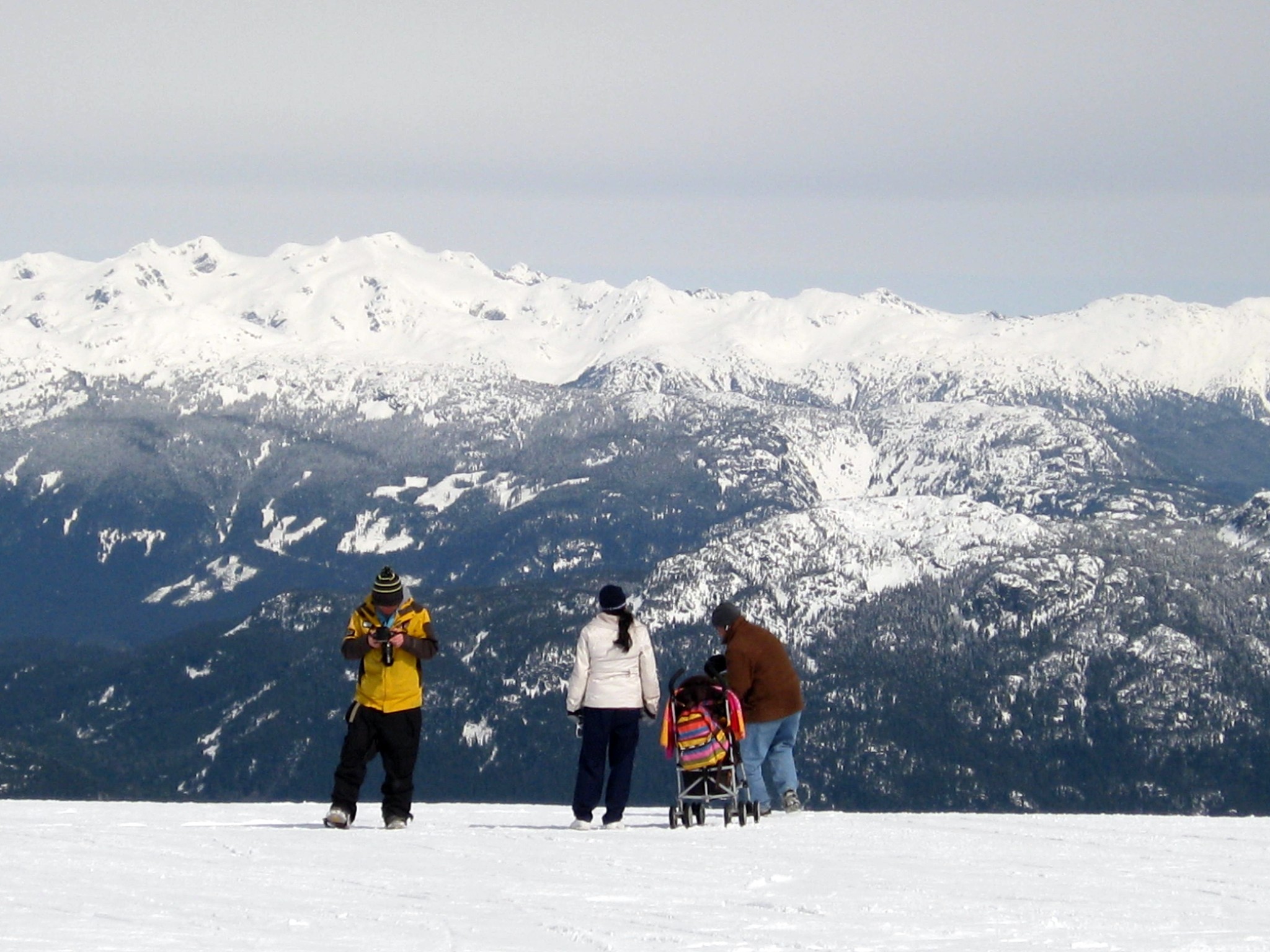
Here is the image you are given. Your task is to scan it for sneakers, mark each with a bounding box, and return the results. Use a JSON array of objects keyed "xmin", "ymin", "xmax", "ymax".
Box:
[
  {"xmin": 784, "ymin": 790, "xmax": 800, "ymax": 813},
  {"xmin": 761, "ymin": 810, "xmax": 772, "ymax": 817}
]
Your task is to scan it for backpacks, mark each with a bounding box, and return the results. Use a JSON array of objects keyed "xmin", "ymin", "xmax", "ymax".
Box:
[{"xmin": 675, "ymin": 703, "xmax": 729, "ymax": 770}]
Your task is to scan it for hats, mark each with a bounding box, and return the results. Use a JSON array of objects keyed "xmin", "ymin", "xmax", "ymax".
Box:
[
  {"xmin": 599, "ymin": 585, "xmax": 626, "ymax": 612},
  {"xmin": 371, "ymin": 566, "xmax": 403, "ymax": 606},
  {"xmin": 712, "ymin": 603, "xmax": 740, "ymax": 626}
]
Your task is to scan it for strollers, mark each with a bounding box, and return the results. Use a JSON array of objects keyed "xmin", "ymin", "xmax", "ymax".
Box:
[{"xmin": 667, "ymin": 667, "xmax": 760, "ymax": 830}]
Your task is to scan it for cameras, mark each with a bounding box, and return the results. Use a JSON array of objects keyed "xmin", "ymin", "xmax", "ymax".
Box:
[{"xmin": 376, "ymin": 626, "xmax": 393, "ymax": 664}]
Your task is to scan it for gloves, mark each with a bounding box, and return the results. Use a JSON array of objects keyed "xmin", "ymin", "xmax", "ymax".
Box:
[
  {"xmin": 567, "ymin": 710, "xmax": 581, "ymax": 724},
  {"xmin": 640, "ymin": 707, "xmax": 656, "ymax": 721}
]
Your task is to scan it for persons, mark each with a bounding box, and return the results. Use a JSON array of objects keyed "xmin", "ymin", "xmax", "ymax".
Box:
[
  {"xmin": 323, "ymin": 567, "xmax": 438, "ymax": 830},
  {"xmin": 713, "ymin": 603, "xmax": 806, "ymax": 817},
  {"xmin": 565, "ymin": 586, "xmax": 661, "ymax": 830}
]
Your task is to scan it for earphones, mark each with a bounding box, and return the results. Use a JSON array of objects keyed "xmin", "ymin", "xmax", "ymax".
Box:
[{"xmin": 725, "ymin": 624, "xmax": 729, "ymax": 631}]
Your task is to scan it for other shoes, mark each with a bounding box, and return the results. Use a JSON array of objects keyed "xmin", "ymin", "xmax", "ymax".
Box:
[
  {"xmin": 603, "ymin": 821, "xmax": 624, "ymax": 829},
  {"xmin": 326, "ymin": 808, "xmax": 351, "ymax": 828},
  {"xmin": 570, "ymin": 819, "xmax": 590, "ymax": 830},
  {"xmin": 387, "ymin": 818, "xmax": 406, "ymax": 829}
]
